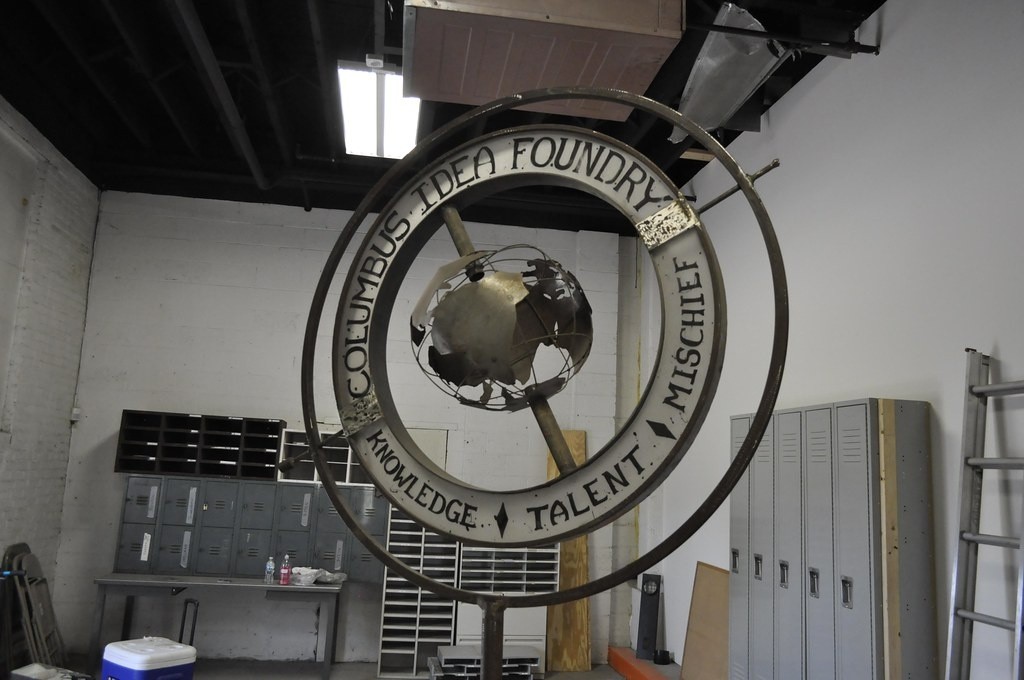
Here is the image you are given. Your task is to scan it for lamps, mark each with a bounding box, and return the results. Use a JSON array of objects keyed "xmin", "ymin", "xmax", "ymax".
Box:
[{"xmin": 337, "ymin": 51, "xmax": 435, "ymax": 160}]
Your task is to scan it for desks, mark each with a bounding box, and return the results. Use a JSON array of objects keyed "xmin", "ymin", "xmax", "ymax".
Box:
[{"xmin": 86, "ymin": 572, "xmax": 344, "ymax": 680}]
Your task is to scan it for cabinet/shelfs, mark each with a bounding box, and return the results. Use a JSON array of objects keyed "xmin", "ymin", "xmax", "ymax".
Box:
[
  {"xmin": 376, "ymin": 503, "xmax": 560, "ymax": 680},
  {"xmin": 112, "ymin": 474, "xmax": 389, "ymax": 585},
  {"xmin": 113, "ymin": 409, "xmax": 287, "ymax": 481},
  {"xmin": 729, "ymin": 398, "xmax": 940, "ymax": 680},
  {"xmin": 277, "ymin": 428, "xmax": 375, "ymax": 487}
]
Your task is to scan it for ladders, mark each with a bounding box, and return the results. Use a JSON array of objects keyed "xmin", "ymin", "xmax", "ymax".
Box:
[{"xmin": 943, "ymin": 344, "xmax": 1024, "ymax": 680}]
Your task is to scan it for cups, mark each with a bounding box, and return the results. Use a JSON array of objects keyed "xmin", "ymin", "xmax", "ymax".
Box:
[{"xmin": 294, "ymin": 567, "xmax": 321, "ymax": 585}]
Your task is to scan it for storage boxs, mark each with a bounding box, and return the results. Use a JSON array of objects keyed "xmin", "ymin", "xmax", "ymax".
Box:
[{"xmin": 101, "ymin": 637, "xmax": 197, "ymax": 680}]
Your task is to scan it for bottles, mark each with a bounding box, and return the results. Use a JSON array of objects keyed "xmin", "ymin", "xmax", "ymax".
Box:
[
  {"xmin": 280, "ymin": 554, "xmax": 291, "ymax": 585},
  {"xmin": 265, "ymin": 557, "xmax": 275, "ymax": 584}
]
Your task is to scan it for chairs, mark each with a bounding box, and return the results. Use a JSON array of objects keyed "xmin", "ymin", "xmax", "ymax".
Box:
[{"xmin": 0, "ymin": 541, "xmax": 69, "ymax": 680}]
